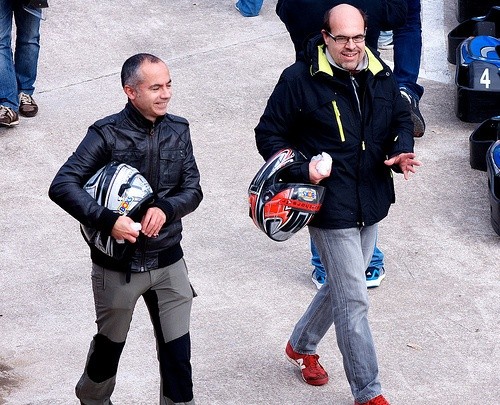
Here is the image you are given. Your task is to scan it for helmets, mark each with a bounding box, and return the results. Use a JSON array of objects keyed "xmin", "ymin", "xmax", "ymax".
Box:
[
  {"xmin": 77, "ymin": 160, "xmax": 159, "ymax": 261},
  {"xmin": 247, "ymin": 147, "xmax": 326, "ymax": 242}
]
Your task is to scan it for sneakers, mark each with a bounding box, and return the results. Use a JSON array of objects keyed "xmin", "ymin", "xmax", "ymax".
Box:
[
  {"xmin": 365, "ymin": 265, "xmax": 386, "ymax": 287},
  {"xmin": 284, "ymin": 339, "xmax": 329, "ymax": 386},
  {"xmin": 17, "ymin": 92, "xmax": 39, "ymax": 117},
  {"xmin": 398, "ymin": 88, "xmax": 425, "ymax": 138},
  {"xmin": 354, "ymin": 393, "xmax": 391, "ymax": 405},
  {"xmin": 0, "ymin": 105, "xmax": 19, "ymax": 126},
  {"xmin": 311, "ymin": 269, "xmax": 326, "ymax": 289}
]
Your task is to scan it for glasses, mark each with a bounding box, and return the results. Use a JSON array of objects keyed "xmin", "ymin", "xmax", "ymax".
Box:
[{"xmin": 326, "ymin": 31, "xmax": 367, "ymax": 44}]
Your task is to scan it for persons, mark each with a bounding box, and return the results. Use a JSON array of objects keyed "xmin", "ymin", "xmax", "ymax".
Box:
[
  {"xmin": 254, "ymin": 4, "xmax": 423, "ymax": 405},
  {"xmin": 233, "ymin": 0, "xmax": 428, "ymax": 289},
  {"xmin": 0, "ymin": 0, "xmax": 49, "ymax": 127},
  {"xmin": 48, "ymin": 53, "xmax": 203, "ymax": 405}
]
